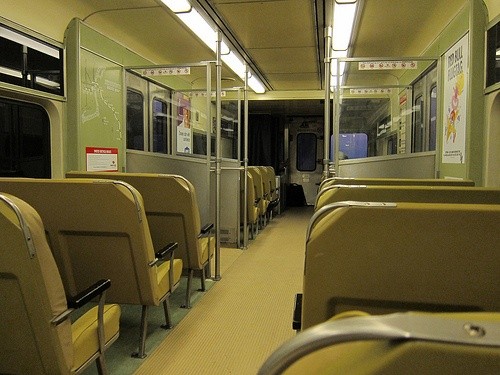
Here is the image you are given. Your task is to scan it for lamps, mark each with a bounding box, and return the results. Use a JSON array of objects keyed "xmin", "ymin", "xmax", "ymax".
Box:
[
  {"xmin": 324, "ymin": 0, "xmax": 365, "ymax": 92},
  {"xmin": 155, "ymin": 0, "xmax": 267, "ymax": 95}
]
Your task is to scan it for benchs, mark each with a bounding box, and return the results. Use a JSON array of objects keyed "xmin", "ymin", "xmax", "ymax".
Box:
[
  {"xmin": 239, "ymin": 165, "xmax": 280, "ymax": 240},
  {"xmin": 258, "ymin": 177, "xmax": 500, "ymax": 375},
  {"xmin": 0, "ymin": 171, "xmax": 215, "ymax": 375}
]
